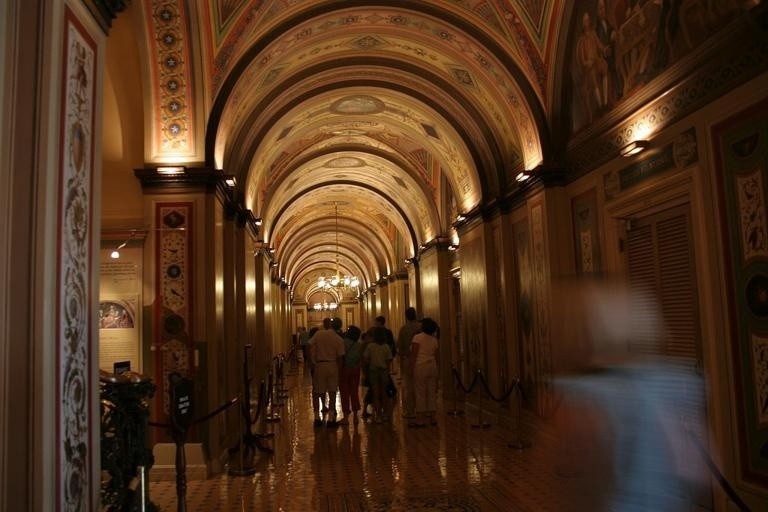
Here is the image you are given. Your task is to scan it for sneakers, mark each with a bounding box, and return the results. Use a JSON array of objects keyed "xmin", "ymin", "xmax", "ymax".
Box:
[
  {"xmin": 313, "ymin": 406, "xmax": 358, "ymax": 429},
  {"xmin": 401, "ymin": 411, "xmax": 437, "ymax": 427},
  {"xmin": 362, "ymin": 410, "xmax": 389, "ymax": 424}
]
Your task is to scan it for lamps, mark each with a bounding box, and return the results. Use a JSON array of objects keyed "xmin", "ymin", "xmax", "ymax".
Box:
[
  {"xmin": 109, "ymin": 231, "xmax": 137, "ymax": 258},
  {"xmin": 313, "ymin": 202, "xmax": 360, "ymax": 311}
]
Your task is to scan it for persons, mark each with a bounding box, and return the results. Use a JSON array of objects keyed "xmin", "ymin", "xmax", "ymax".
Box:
[{"xmin": 297, "ymin": 307, "xmax": 440, "ymax": 429}]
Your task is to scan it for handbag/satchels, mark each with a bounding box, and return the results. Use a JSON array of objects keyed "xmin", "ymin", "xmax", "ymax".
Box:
[{"xmin": 377, "ymin": 366, "xmax": 390, "ymax": 385}]
